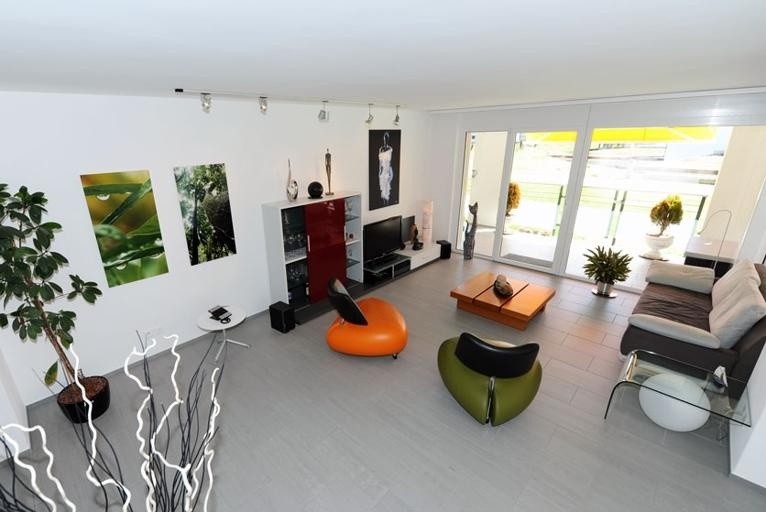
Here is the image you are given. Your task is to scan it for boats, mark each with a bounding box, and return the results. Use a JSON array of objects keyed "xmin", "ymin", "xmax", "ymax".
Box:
[
  {"xmin": 269, "ymin": 300, "xmax": 296, "ymax": 334},
  {"xmin": 436, "ymin": 239, "xmax": 452, "ymax": 260}
]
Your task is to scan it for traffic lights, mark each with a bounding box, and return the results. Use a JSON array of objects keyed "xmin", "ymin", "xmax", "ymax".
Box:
[
  {"xmin": 711, "ymin": 258, "xmax": 761, "ymax": 308},
  {"xmin": 708, "ymin": 278, "xmax": 765, "ymax": 350}
]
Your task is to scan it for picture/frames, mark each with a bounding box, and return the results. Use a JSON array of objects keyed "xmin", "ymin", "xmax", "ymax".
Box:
[
  {"xmin": 448, "ymin": 270, "xmax": 557, "ymax": 332},
  {"xmin": 602, "ymin": 347, "xmax": 751, "ymax": 428}
]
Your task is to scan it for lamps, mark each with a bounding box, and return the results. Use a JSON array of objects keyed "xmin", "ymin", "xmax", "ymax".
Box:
[
  {"xmin": 437, "ymin": 332, "xmax": 543, "ymax": 427},
  {"xmin": 326, "ymin": 297, "xmax": 408, "ymax": 360}
]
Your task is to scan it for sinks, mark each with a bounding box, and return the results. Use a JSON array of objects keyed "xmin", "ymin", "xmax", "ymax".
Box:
[{"xmin": 618, "ymin": 252, "xmax": 765, "ymax": 400}]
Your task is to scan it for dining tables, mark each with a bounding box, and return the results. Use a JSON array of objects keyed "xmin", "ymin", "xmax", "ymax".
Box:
[
  {"xmin": 0, "ymin": 183, "xmax": 110, "ymax": 424},
  {"xmin": 491, "ymin": 182, "xmax": 522, "ymax": 235},
  {"xmin": 581, "ymin": 243, "xmax": 634, "ymax": 298},
  {"xmin": 638, "ymin": 191, "xmax": 683, "ymax": 262}
]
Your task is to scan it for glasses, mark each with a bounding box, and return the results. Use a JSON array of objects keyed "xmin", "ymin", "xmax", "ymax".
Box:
[
  {"xmin": 365, "ymin": 103, "xmax": 376, "ymax": 123},
  {"xmin": 422, "ymin": 199, "xmax": 433, "ymax": 243},
  {"xmin": 393, "ymin": 105, "xmax": 401, "ymax": 127},
  {"xmin": 199, "ymin": 92, "xmax": 212, "ymax": 113},
  {"xmin": 258, "ymin": 96, "xmax": 268, "ymax": 114},
  {"xmin": 318, "ymin": 100, "xmax": 329, "ymax": 122}
]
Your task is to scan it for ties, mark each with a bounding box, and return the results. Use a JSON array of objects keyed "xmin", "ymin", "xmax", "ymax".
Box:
[{"xmin": 363, "ymin": 215, "xmax": 403, "ymax": 266}]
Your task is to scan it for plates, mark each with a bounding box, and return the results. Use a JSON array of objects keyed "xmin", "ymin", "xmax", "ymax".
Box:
[
  {"xmin": 393, "ymin": 240, "xmax": 441, "ymax": 271},
  {"xmin": 261, "ymin": 190, "xmax": 363, "ymax": 310},
  {"xmin": 362, "ymin": 253, "xmax": 411, "ymax": 290}
]
